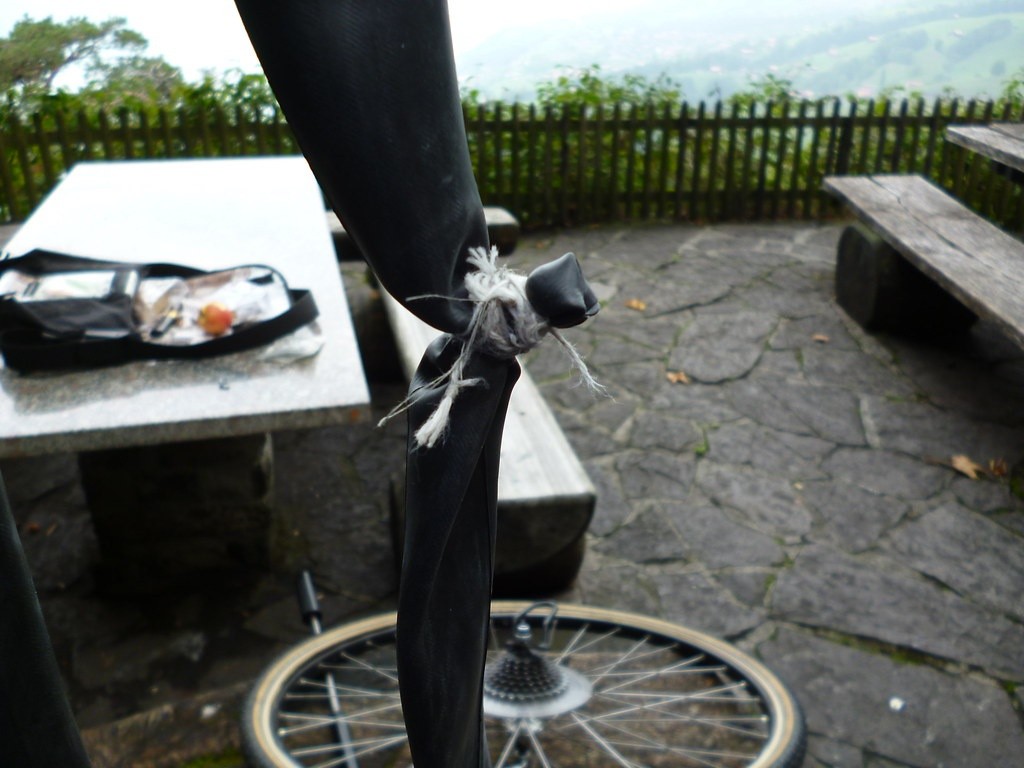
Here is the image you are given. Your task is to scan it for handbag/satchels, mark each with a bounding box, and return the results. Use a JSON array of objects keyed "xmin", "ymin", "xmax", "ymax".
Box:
[{"xmin": 0, "ymin": 249, "xmax": 316, "ymax": 378}]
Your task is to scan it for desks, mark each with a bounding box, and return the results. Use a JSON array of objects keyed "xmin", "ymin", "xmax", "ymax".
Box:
[
  {"xmin": 946, "ymin": 122, "xmax": 1024, "ymax": 173},
  {"xmin": 0, "ymin": 156, "xmax": 374, "ymax": 628}
]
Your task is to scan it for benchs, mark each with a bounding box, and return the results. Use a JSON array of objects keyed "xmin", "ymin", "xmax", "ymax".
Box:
[
  {"xmin": 818, "ymin": 172, "xmax": 1024, "ymax": 353},
  {"xmin": 367, "ymin": 265, "xmax": 595, "ymax": 596},
  {"xmin": 327, "ymin": 207, "xmax": 516, "ymax": 262}
]
